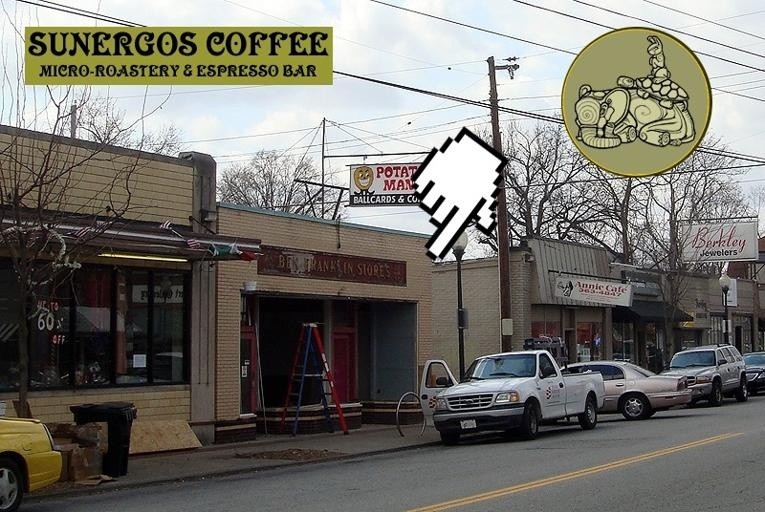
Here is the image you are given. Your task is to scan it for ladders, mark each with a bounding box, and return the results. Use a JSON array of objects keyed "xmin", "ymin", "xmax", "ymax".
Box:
[{"xmin": 279, "ymin": 322, "xmax": 350, "ymax": 437}]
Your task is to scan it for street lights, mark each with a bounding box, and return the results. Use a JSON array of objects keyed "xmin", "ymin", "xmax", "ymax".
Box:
[
  {"xmin": 719, "ymin": 271, "xmax": 732, "ymax": 344},
  {"xmin": 451, "ymin": 230, "xmax": 469, "ymax": 382}
]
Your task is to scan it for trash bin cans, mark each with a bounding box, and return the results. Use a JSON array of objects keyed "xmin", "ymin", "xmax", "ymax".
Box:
[{"xmin": 69, "ymin": 401, "xmax": 137, "ymax": 478}]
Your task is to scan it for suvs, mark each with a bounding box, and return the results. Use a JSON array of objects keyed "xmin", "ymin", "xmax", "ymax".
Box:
[{"xmin": 659, "ymin": 342, "xmax": 748, "ymax": 406}]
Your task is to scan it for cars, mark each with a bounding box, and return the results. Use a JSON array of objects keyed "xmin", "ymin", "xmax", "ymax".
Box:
[
  {"xmin": 742, "ymin": 352, "xmax": 765, "ymax": 396},
  {"xmin": 0, "ymin": 414, "xmax": 65, "ymax": 511},
  {"xmin": 559, "ymin": 359, "xmax": 694, "ymax": 421},
  {"xmin": 117, "ymin": 350, "xmax": 186, "ymax": 384}
]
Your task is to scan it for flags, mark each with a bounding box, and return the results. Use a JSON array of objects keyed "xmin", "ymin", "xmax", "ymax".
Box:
[
  {"xmin": 211, "ymin": 244, "xmax": 230, "ymax": 256},
  {"xmin": 186, "ymin": 237, "xmax": 200, "ymax": 248},
  {"xmin": 160, "ymin": 218, "xmax": 172, "ymax": 231},
  {"xmin": 237, "ymin": 250, "xmax": 257, "ymax": 260}
]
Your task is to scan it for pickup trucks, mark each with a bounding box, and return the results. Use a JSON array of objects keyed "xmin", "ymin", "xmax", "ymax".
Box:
[{"xmin": 419, "ymin": 348, "xmax": 607, "ymax": 447}]
